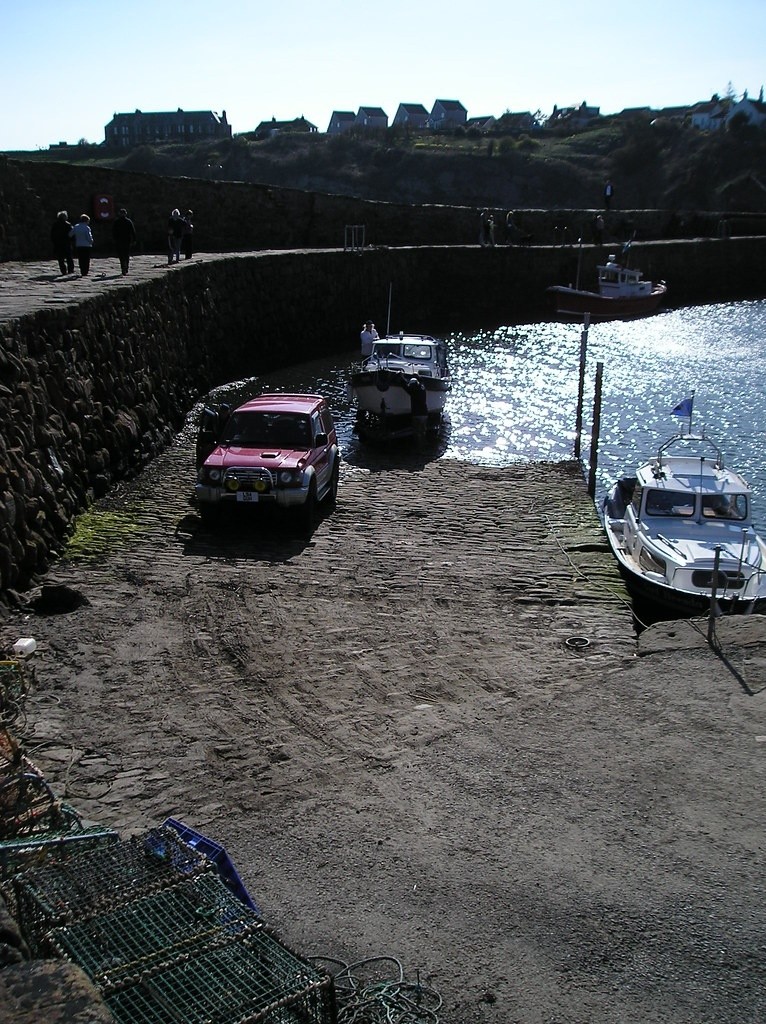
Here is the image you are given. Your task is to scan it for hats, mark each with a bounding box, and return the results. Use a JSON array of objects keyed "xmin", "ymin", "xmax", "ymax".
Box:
[{"xmin": 366, "ymin": 320, "xmax": 373, "ymax": 325}]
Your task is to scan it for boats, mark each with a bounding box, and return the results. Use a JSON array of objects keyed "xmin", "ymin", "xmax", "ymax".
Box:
[
  {"xmin": 544, "ymin": 235, "xmax": 669, "ymax": 321},
  {"xmin": 603, "ymin": 426, "xmax": 766, "ymax": 618},
  {"xmin": 349, "ymin": 279, "xmax": 452, "ymax": 433}
]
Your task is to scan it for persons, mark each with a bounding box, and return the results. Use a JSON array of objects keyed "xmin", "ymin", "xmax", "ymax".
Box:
[
  {"xmin": 594, "ymin": 215, "xmax": 604, "ymax": 246},
  {"xmin": 205, "ymin": 404, "xmax": 235, "ymax": 433},
  {"xmin": 167, "ymin": 209, "xmax": 194, "ymax": 264},
  {"xmin": 479, "ymin": 208, "xmax": 514, "ymax": 247},
  {"xmin": 605, "ymin": 254, "xmax": 622, "ymax": 281},
  {"xmin": 603, "ymin": 180, "xmax": 614, "ymax": 211},
  {"xmin": 360, "ymin": 320, "xmax": 378, "ymax": 360},
  {"xmin": 111, "ymin": 208, "xmax": 136, "ymax": 276},
  {"xmin": 50, "ymin": 211, "xmax": 93, "ymax": 276}
]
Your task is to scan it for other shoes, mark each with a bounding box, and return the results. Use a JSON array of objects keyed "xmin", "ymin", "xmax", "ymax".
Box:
[
  {"xmin": 63, "ymin": 272, "xmax": 68, "ymax": 275},
  {"xmin": 68, "ymin": 271, "xmax": 75, "ymax": 274}
]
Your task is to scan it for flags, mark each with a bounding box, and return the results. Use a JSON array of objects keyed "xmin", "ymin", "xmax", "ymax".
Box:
[{"xmin": 669, "ymin": 399, "xmax": 693, "ymax": 416}]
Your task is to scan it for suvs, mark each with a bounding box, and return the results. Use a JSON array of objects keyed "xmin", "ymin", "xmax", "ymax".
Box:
[{"xmin": 195, "ymin": 392, "xmax": 342, "ymax": 531}]
